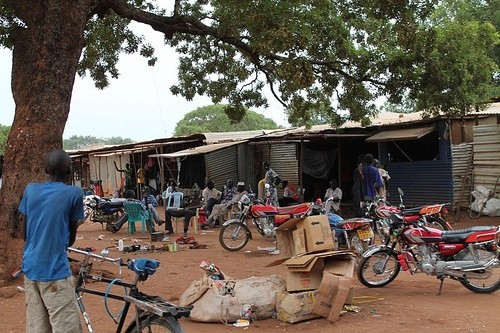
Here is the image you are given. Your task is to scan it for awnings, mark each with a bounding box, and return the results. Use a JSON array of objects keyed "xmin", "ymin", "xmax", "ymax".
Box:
[{"xmin": 365, "ymin": 125, "xmax": 435, "ymax": 140}]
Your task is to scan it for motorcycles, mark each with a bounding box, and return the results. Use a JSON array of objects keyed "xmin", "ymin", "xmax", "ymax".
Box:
[
  {"xmin": 75, "ymin": 180, "xmax": 133, "ymax": 231},
  {"xmin": 360, "ymin": 173, "xmax": 454, "ymax": 246},
  {"xmin": 218, "ymin": 185, "xmax": 323, "ymax": 252},
  {"xmin": 356, "ymin": 187, "xmax": 500, "ymax": 294},
  {"xmin": 309, "ymin": 196, "xmax": 374, "ymax": 271}
]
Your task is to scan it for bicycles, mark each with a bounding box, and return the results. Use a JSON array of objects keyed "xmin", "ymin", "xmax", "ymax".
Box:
[{"xmin": 66, "ymin": 246, "xmax": 194, "ymax": 333}]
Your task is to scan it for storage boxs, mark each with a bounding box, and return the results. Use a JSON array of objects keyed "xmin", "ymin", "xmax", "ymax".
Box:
[
  {"xmin": 151, "ymin": 232, "xmax": 164, "ymax": 242},
  {"xmin": 264, "ymin": 215, "xmax": 357, "ymax": 323}
]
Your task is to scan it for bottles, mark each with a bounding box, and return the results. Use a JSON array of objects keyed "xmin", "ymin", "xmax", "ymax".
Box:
[{"xmin": 119, "ymin": 238, "xmax": 124, "ymax": 251}]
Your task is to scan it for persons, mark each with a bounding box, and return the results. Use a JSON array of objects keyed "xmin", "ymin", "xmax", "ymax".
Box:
[
  {"xmin": 218, "ymin": 179, "xmax": 237, "ymax": 204},
  {"xmin": 113, "ymin": 160, "xmax": 135, "ymax": 191},
  {"xmin": 98, "ymin": 180, "xmax": 105, "ymax": 197},
  {"xmin": 202, "ymin": 180, "xmax": 222, "ymax": 225},
  {"xmin": 257, "ymin": 160, "xmax": 299, "ymax": 207},
  {"xmin": 19, "ymin": 150, "xmax": 84, "ymax": 333},
  {"xmin": 86, "ymin": 180, "xmax": 94, "ymax": 191},
  {"xmin": 137, "ymin": 162, "xmax": 145, "ymax": 190},
  {"xmin": 200, "ymin": 182, "xmax": 248, "ymax": 226},
  {"xmin": 73, "ymin": 161, "xmax": 80, "ymax": 180},
  {"xmin": 324, "ymin": 179, "xmax": 342, "ymax": 214},
  {"xmin": 353, "ymin": 154, "xmax": 390, "ymax": 211},
  {"xmin": 107, "ymin": 180, "xmax": 203, "ymax": 234},
  {"xmin": 94, "ymin": 179, "xmax": 100, "ymax": 195}
]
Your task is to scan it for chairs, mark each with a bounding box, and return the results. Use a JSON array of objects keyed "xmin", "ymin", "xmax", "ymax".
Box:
[
  {"xmin": 171, "ymin": 204, "xmax": 243, "ymax": 235},
  {"xmin": 166, "ymin": 192, "xmax": 184, "ymax": 210},
  {"xmin": 124, "ymin": 203, "xmax": 153, "ymax": 236}
]
